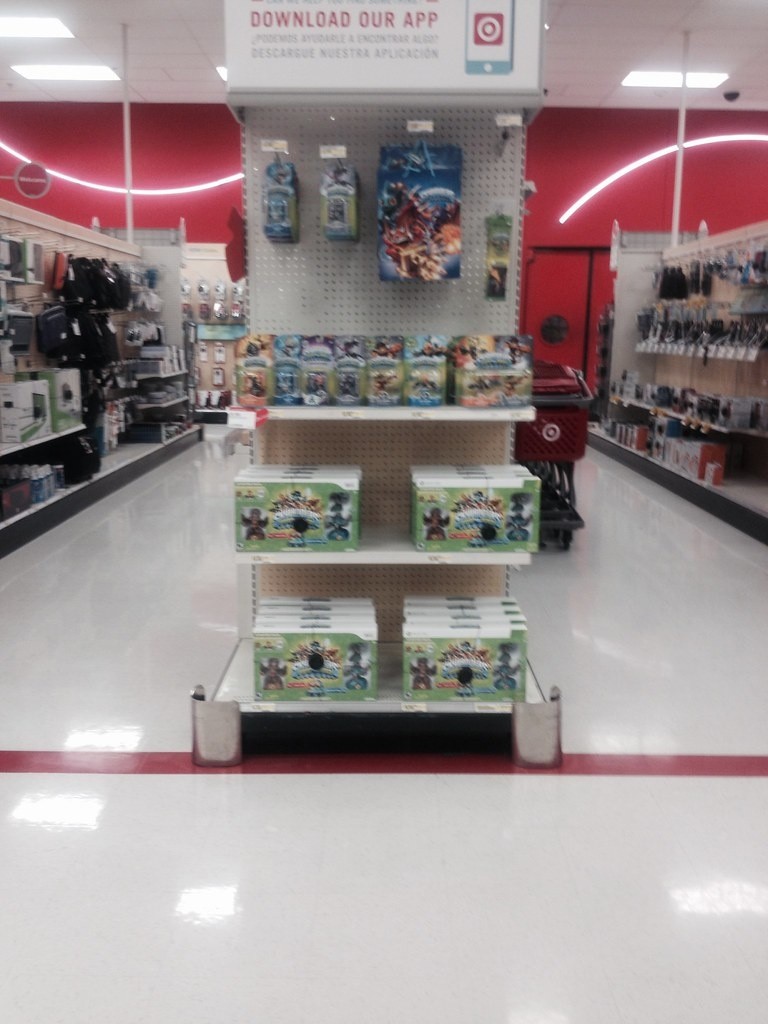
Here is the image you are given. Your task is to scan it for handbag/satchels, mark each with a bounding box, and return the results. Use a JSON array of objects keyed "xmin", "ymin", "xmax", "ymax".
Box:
[{"xmin": 37, "ymin": 303, "xmax": 120, "ymax": 414}]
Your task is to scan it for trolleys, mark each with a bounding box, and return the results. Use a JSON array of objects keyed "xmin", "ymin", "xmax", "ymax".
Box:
[{"xmin": 514, "ymin": 360, "xmax": 593, "ymax": 551}]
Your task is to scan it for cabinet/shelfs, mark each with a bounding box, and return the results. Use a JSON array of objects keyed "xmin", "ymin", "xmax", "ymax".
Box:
[
  {"xmin": 191, "ymin": 87, "xmax": 564, "ymax": 776},
  {"xmin": 585, "ymin": 217, "xmax": 768, "ymax": 549},
  {"xmin": 0, "ymin": 196, "xmax": 205, "ymax": 559}
]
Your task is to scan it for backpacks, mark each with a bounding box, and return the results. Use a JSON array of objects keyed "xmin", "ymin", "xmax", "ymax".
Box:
[{"xmin": 53, "ymin": 254, "xmax": 131, "ymax": 310}]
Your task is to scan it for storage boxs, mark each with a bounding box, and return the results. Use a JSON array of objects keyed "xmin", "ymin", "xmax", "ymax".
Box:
[{"xmin": 235, "ymin": 463, "xmax": 542, "ymax": 706}]
